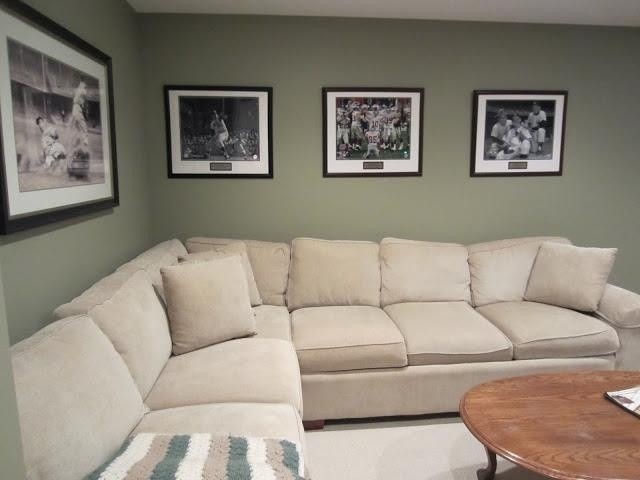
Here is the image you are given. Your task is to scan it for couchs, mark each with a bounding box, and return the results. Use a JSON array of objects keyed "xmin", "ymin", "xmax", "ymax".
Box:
[
  {"xmin": 4, "ymin": 237, "xmax": 313, "ymax": 479},
  {"xmin": 288, "ymin": 234, "xmax": 640, "ymax": 432}
]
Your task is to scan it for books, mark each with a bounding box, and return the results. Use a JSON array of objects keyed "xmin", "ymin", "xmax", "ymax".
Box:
[{"xmin": 604, "ymin": 386, "xmax": 640, "ymax": 416}]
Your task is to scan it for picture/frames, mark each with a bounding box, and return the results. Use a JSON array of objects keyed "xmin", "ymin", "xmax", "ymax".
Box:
[
  {"xmin": 162, "ymin": 84, "xmax": 274, "ymax": 180},
  {"xmin": 468, "ymin": 88, "xmax": 570, "ymax": 179},
  {"xmin": 1, "ymin": 0, "xmax": 121, "ymax": 237},
  {"xmin": 321, "ymin": 85, "xmax": 426, "ymax": 179}
]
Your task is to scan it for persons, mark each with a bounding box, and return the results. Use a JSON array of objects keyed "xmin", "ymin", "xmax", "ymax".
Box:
[
  {"xmin": 524, "ymin": 102, "xmax": 546, "ymax": 154},
  {"xmin": 36, "ymin": 116, "xmax": 67, "ymax": 170},
  {"xmin": 495, "ymin": 112, "xmax": 532, "ymax": 160},
  {"xmin": 488, "ymin": 110, "xmax": 512, "ymax": 158},
  {"xmin": 68, "ymin": 82, "xmax": 88, "ymax": 152},
  {"xmin": 207, "ymin": 109, "xmax": 229, "ymax": 161},
  {"xmin": 336, "ymin": 100, "xmax": 411, "ymax": 159}
]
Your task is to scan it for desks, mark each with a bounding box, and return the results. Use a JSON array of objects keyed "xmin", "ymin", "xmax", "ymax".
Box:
[{"xmin": 456, "ymin": 369, "xmax": 640, "ymax": 480}]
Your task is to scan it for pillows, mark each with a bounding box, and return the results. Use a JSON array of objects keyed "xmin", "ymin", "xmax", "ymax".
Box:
[
  {"xmin": 158, "ymin": 253, "xmax": 258, "ymax": 357},
  {"xmin": 520, "ymin": 240, "xmax": 619, "ymax": 313},
  {"xmin": 177, "ymin": 239, "xmax": 263, "ymax": 307}
]
